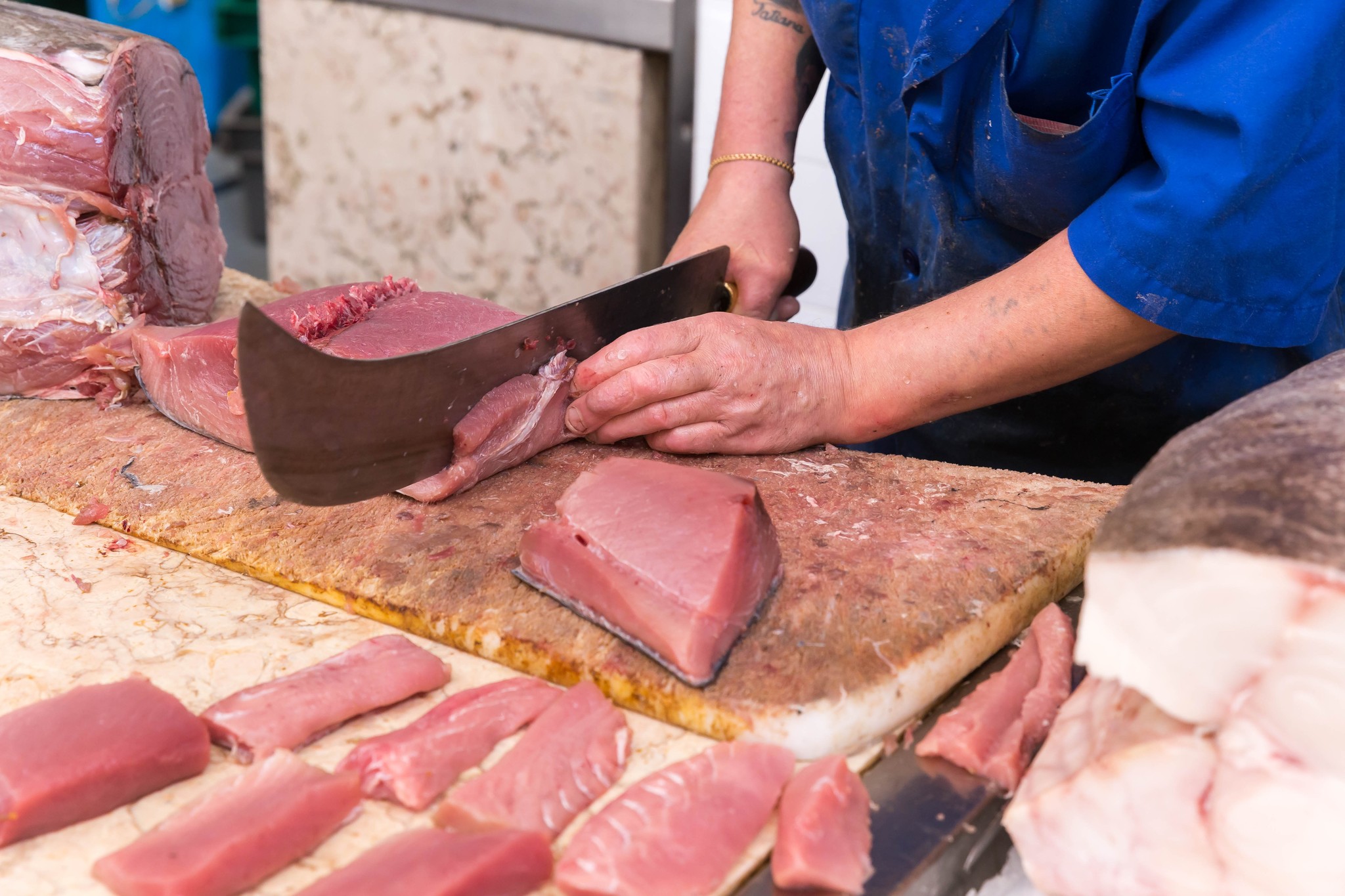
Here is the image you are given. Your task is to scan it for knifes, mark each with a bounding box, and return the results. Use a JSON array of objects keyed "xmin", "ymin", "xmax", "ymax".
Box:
[{"xmin": 238, "ymin": 244, "xmax": 817, "ymax": 506}]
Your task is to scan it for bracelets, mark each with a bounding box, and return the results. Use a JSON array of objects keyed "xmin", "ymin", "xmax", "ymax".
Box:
[{"xmin": 708, "ymin": 154, "xmax": 795, "ymax": 179}]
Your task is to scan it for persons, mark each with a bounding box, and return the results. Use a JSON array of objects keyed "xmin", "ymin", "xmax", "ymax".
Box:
[{"xmin": 565, "ymin": 0, "xmax": 1345, "ymax": 490}]
